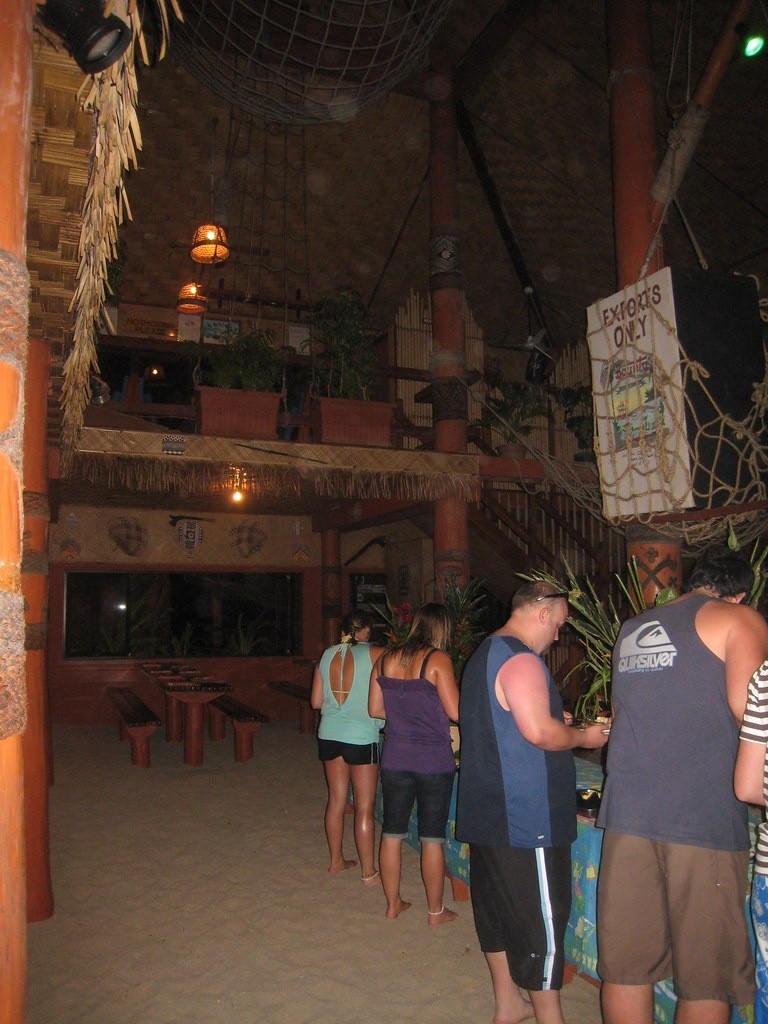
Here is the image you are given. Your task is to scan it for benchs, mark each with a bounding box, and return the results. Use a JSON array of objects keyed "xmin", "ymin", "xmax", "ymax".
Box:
[
  {"xmin": 105, "ymin": 687, "xmax": 163, "ymax": 769},
  {"xmin": 268, "ymin": 681, "xmax": 315, "ymax": 738},
  {"xmin": 208, "ymin": 695, "xmax": 271, "ymax": 764}
]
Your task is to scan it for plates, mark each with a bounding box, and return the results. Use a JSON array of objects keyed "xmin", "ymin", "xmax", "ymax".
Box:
[{"xmin": 579, "ymin": 727, "xmax": 611, "ymax": 736}]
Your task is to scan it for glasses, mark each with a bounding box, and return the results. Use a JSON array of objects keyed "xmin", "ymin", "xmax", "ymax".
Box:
[{"xmin": 538, "ymin": 592, "xmax": 569, "ymax": 602}]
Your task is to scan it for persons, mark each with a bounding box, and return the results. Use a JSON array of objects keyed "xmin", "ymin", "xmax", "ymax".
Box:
[
  {"xmin": 312, "ymin": 612, "xmax": 382, "ymax": 888},
  {"xmin": 732, "ymin": 656, "xmax": 767, "ymax": 1024},
  {"xmin": 367, "ymin": 602, "xmax": 461, "ymax": 925},
  {"xmin": 595, "ymin": 543, "xmax": 766, "ymax": 1024},
  {"xmin": 455, "ymin": 580, "xmax": 609, "ymax": 1024}
]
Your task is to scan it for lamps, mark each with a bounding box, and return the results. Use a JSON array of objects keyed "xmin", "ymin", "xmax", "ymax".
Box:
[
  {"xmin": 47, "ymin": 1, "xmax": 134, "ymax": 76},
  {"xmin": 733, "ymin": 22, "xmax": 764, "ymax": 58},
  {"xmin": 175, "ymin": 267, "xmax": 209, "ymax": 316},
  {"xmin": 189, "ymin": 118, "xmax": 231, "ymax": 266}
]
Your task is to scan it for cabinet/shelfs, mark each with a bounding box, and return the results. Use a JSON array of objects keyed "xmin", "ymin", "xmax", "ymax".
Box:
[{"xmin": 351, "ymin": 574, "xmax": 390, "ymax": 646}]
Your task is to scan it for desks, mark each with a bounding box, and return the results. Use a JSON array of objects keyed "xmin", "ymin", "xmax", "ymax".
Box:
[{"xmin": 138, "ymin": 662, "xmax": 234, "ymax": 765}]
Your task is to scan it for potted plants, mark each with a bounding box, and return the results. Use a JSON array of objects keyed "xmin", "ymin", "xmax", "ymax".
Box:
[
  {"xmin": 474, "ymin": 382, "xmax": 554, "ymax": 458},
  {"xmin": 188, "ymin": 331, "xmax": 293, "ymax": 441},
  {"xmin": 300, "ymin": 288, "xmax": 396, "ymax": 448}
]
